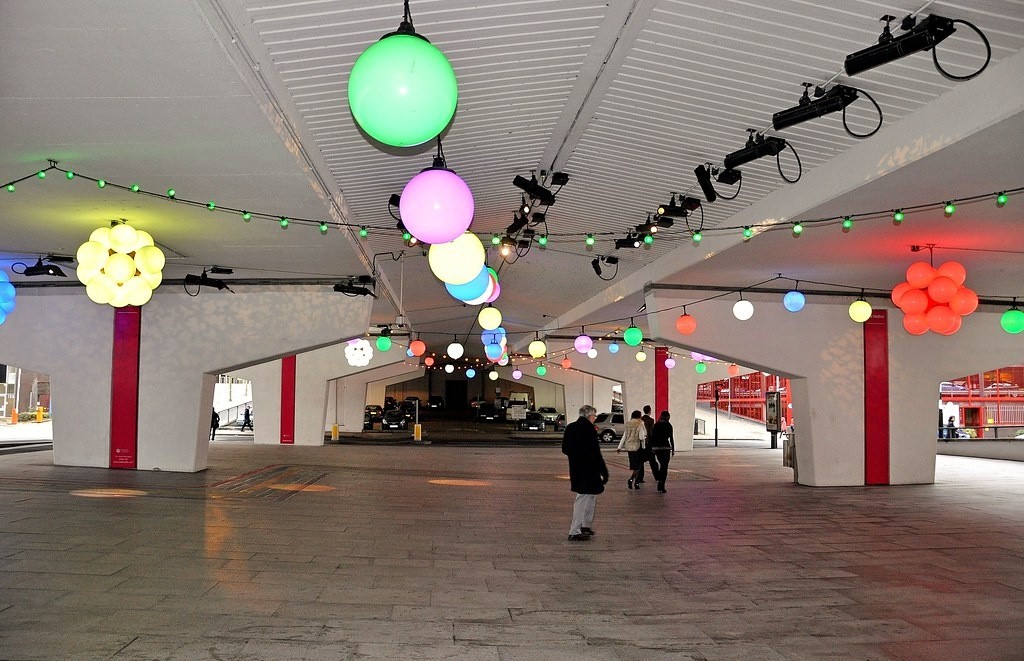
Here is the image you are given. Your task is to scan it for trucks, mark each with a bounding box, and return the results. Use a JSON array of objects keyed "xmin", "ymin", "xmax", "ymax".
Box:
[{"xmin": 506, "ymin": 392, "xmax": 530, "ymax": 424}]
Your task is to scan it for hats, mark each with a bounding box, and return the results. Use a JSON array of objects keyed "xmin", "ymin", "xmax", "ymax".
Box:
[{"xmin": 658, "ymin": 410, "xmax": 670, "ymax": 422}]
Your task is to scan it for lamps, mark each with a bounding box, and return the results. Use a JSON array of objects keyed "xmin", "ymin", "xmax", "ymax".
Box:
[
  {"xmin": 503, "ymin": 14, "xmax": 957, "ymax": 276},
  {"xmin": 23, "ymin": 257, "xmax": 67, "ymax": 276},
  {"xmin": 333, "ymin": 283, "xmax": 378, "ymax": 299},
  {"xmin": 185, "ymin": 275, "xmax": 226, "ymax": 291},
  {"xmin": 387, "ymin": 195, "xmax": 401, "ymax": 210}
]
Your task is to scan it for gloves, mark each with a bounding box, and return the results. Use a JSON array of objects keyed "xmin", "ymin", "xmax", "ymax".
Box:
[{"xmin": 602, "ymin": 475, "xmax": 607, "ymax": 485}]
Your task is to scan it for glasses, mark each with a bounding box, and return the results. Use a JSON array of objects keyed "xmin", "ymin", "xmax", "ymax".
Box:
[{"xmin": 590, "ymin": 413, "xmax": 597, "ymax": 419}]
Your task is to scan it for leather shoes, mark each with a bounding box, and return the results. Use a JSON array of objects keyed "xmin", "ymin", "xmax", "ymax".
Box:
[
  {"xmin": 580, "ymin": 526, "xmax": 595, "ymax": 536},
  {"xmin": 568, "ymin": 533, "xmax": 588, "ymax": 542}
]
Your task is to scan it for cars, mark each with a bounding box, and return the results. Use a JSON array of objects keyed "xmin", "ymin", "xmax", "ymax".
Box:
[
  {"xmin": 364, "ymin": 410, "xmax": 374, "ymax": 430},
  {"xmin": 941, "ymin": 382, "xmax": 967, "ymax": 392},
  {"xmin": 518, "ymin": 412, "xmax": 546, "ymax": 432},
  {"xmin": 384, "ymin": 396, "xmax": 422, "ymax": 422},
  {"xmin": 593, "ymin": 412, "xmax": 625, "ymax": 443},
  {"xmin": 984, "ymin": 383, "xmax": 1018, "ymax": 397},
  {"xmin": 381, "ymin": 410, "xmax": 408, "ymax": 430},
  {"xmin": 537, "ymin": 407, "xmax": 559, "ymax": 425},
  {"xmin": 366, "ymin": 405, "xmax": 383, "ymax": 422},
  {"xmin": 426, "ymin": 396, "xmax": 445, "ymax": 410},
  {"xmin": 554, "ymin": 414, "xmax": 566, "ymax": 432},
  {"xmin": 468, "ymin": 397, "xmax": 509, "ymax": 424},
  {"xmin": 937, "ymin": 424, "xmax": 970, "ymax": 439}
]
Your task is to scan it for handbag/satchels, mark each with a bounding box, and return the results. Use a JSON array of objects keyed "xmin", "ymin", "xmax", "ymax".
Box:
[{"xmin": 637, "ymin": 440, "xmax": 650, "ymax": 463}]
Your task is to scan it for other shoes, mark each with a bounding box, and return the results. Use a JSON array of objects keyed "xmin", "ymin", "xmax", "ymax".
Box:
[
  {"xmin": 634, "ymin": 483, "xmax": 640, "ymax": 490},
  {"xmin": 628, "ymin": 479, "xmax": 632, "ymax": 489},
  {"xmin": 660, "ymin": 490, "xmax": 666, "ymax": 494},
  {"xmin": 639, "ymin": 480, "xmax": 646, "ymax": 483}
]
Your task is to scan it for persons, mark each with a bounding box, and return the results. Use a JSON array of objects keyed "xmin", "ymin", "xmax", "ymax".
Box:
[
  {"xmin": 946, "ymin": 416, "xmax": 958, "ymax": 438},
  {"xmin": 617, "ymin": 404, "xmax": 675, "ymax": 493},
  {"xmin": 779, "ymin": 417, "xmax": 789, "ymax": 439},
  {"xmin": 241, "ymin": 406, "xmax": 253, "ymax": 432},
  {"xmin": 210, "ymin": 407, "xmax": 220, "ymax": 441},
  {"xmin": 562, "ymin": 405, "xmax": 609, "ymax": 541}
]
